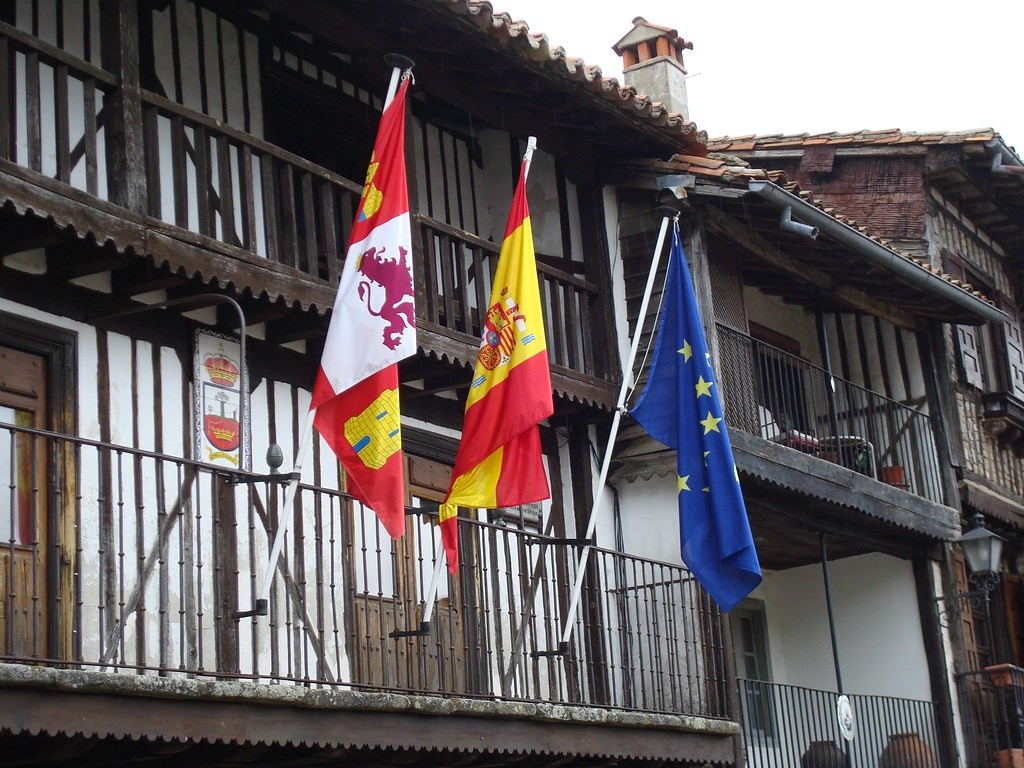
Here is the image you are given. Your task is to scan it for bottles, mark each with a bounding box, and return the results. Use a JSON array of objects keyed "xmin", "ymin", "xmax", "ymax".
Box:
[
  {"xmin": 879, "ymin": 732, "xmax": 941, "ymax": 768},
  {"xmin": 801, "ymin": 741, "xmax": 852, "ymax": 768}
]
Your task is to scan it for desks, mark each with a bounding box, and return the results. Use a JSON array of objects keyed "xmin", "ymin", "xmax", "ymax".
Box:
[{"xmin": 818, "ymin": 436, "xmax": 878, "ymax": 480}]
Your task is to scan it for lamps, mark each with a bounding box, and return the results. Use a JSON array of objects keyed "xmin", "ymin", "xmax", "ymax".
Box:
[{"xmin": 933, "ymin": 512, "xmax": 1009, "ymax": 629}]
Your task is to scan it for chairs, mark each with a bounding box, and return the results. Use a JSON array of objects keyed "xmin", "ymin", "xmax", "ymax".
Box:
[
  {"xmin": 881, "ymin": 465, "xmax": 909, "ymax": 491},
  {"xmin": 759, "ymin": 405, "xmax": 818, "ymax": 454}
]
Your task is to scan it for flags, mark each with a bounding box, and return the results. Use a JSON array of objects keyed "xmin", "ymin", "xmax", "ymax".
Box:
[
  {"xmin": 625, "ymin": 225, "xmax": 763, "ymax": 612},
  {"xmin": 437, "ymin": 162, "xmax": 554, "ymax": 576},
  {"xmin": 307, "ymin": 79, "xmax": 417, "ymax": 539}
]
